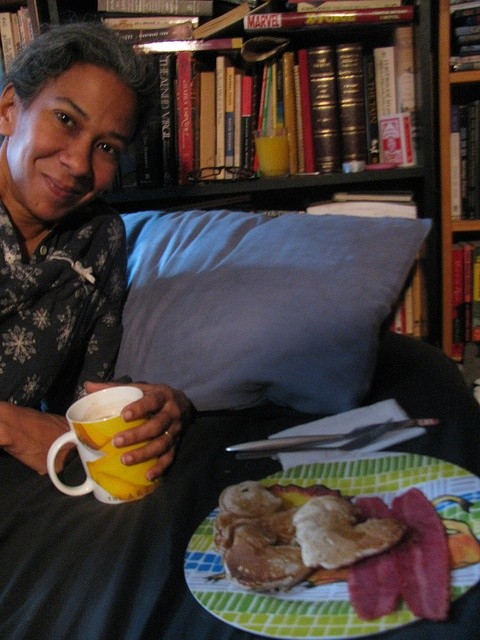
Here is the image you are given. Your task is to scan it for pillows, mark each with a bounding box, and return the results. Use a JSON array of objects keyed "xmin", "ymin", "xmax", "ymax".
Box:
[{"xmin": 114, "ymin": 210, "xmax": 432, "ymax": 419}]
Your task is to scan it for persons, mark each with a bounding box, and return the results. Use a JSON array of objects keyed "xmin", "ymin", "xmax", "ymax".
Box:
[{"xmin": 1, "ymin": 22, "xmax": 183, "ymax": 482}]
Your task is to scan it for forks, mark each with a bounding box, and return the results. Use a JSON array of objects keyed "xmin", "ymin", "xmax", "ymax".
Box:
[{"xmin": 234, "ymin": 419, "xmax": 395, "ymax": 463}]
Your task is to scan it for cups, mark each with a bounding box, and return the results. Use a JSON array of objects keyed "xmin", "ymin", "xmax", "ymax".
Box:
[
  {"xmin": 252, "ymin": 127, "xmax": 291, "ymax": 181},
  {"xmin": 48, "ymin": 387, "xmax": 166, "ymax": 505}
]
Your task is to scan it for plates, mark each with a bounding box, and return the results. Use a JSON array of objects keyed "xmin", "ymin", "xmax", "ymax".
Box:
[{"xmin": 184, "ymin": 452, "xmax": 479, "ymax": 639}]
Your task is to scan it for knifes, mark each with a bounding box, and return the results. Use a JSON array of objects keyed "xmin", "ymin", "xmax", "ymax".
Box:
[{"xmin": 222, "ymin": 418, "xmax": 440, "ymax": 448}]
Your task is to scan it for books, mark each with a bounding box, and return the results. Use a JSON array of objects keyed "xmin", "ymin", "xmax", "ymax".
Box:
[
  {"xmin": 115, "ymin": 54, "xmax": 175, "ymax": 186},
  {"xmin": 97, "ymin": 0, "xmax": 251, "ymax": 52},
  {"xmin": 177, "ymin": 27, "xmax": 417, "ymax": 183},
  {"xmin": 1, "ymin": 6, "xmax": 35, "ymax": 74},
  {"xmin": 450, "ymin": 1, "xmax": 479, "ymax": 72},
  {"xmin": 243, "ymin": 0, "xmax": 414, "ymax": 30},
  {"xmin": 450, "ymin": 99, "xmax": 479, "ymax": 219},
  {"xmin": 386, "ymin": 246, "xmax": 428, "ymax": 340},
  {"xmin": 305, "ymin": 193, "xmax": 419, "ymax": 219},
  {"xmin": 451, "ymin": 238, "xmax": 480, "ymax": 362}
]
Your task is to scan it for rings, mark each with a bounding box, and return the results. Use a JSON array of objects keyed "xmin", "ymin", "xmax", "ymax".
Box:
[{"xmin": 164, "ymin": 431, "xmax": 175, "ymax": 442}]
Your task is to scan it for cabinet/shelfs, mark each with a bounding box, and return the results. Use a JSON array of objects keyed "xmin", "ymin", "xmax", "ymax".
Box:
[
  {"xmin": 435, "ymin": 0, "xmax": 479, "ymax": 363},
  {"xmin": 48, "ymin": 0, "xmax": 441, "ymax": 353}
]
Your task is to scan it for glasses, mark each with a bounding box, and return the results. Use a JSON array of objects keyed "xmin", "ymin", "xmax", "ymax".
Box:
[{"xmin": 187, "ymin": 165, "xmax": 257, "ymax": 184}]
